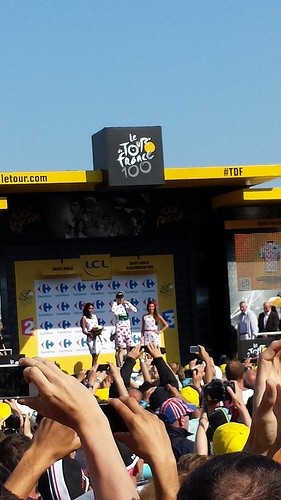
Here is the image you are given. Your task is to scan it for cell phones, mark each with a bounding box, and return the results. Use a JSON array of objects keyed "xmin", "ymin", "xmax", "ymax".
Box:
[
  {"xmin": 99, "ymin": 404, "xmax": 129, "ymax": 433},
  {"xmin": 97, "ymin": 364, "xmax": 109, "ymax": 371},
  {"xmin": 0, "ymin": 364, "xmax": 39, "ymax": 400},
  {"xmin": 190, "ymin": 346, "xmax": 199, "ymax": 353},
  {"xmin": 140, "ymin": 346, "xmax": 149, "ymax": 352}
]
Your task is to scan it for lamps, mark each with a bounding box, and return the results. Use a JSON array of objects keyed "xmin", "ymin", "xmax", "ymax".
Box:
[
  {"xmin": 21, "ymin": 289, "xmax": 34, "ymax": 301},
  {"xmin": 160, "ymin": 282, "xmax": 175, "ymax": 296}
]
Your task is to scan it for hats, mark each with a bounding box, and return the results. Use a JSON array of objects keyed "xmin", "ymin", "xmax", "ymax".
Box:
[
  {"xmin": 160, "ymin": 397, "xmax": 196, "ymax": 423},
  {"xmin": 214, "ymin": 366, "xmax": 222, "ymax": 379},
  {"xmin": 213, "ymin": 422, "xmax": 249, "ymax": 455},
  {"xmin": 114, "ymin": 439, "xmax": 139, "ymax": 469},
  {"xmin": 179, "ymin": 385, "xmax": 200, "ymax": 408},
  {"xmin": 220, "ymin": 363, "xmax": 227, "ymax": 373},
  {"xmin": 37, "ymin": 457, "xmax": 89, "ymax": 500},
  {"xmin": 116, "ymin": 292, "xmax": 123, "ymax": 298},
  {"xmin": 0, "ymin": 403, "xmax": 11, "ymax": 424},
  {"xmin": 208, "ymin": 406, "xmax": 231, "ymax": 435}
]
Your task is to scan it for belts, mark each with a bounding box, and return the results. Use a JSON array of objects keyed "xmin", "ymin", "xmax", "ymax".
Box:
[{"xmin": 242, "ymin": 332, "xmax": 247, "ymax": 335}]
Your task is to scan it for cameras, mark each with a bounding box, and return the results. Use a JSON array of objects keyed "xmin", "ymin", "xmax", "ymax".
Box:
[
  {"xmin": 185, "ymin": 369, "xmax": 198, "ymax": 378},
  {"xmin": 205, "ymin": 379, "xmax": 235, "ymax": 402}
]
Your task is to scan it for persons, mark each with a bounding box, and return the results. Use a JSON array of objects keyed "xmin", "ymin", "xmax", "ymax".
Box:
[
  {"xmin": 258, "ymin": 301, "xmax": 279, "ymax": 333},
  {"xmin": 0, "ymin": 339, "xmax": 281, "ymax": 500},
  {"xmin": 80, "ymin": 303, "xmax": 102, "ymax": 366},
  {"xmin": 110, "ymin": 292, "xmax": 138, "ymax": 373},
  {"xmin": 236, "ymin": 301, "xmax": 259, "ymax": 339},
  {"xmin": 141, "ymin": 300, "xmax": 169, "ymax": 345}
]
